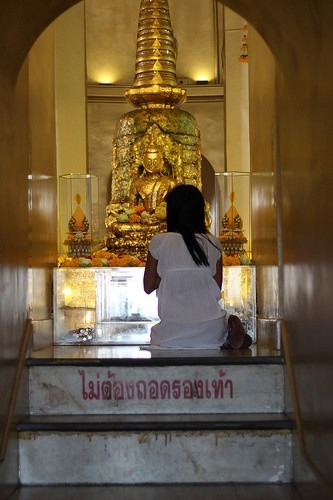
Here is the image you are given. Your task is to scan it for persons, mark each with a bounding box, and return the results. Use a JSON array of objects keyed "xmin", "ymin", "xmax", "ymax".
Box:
[
  {"xmin": 142, "ymin": 185, "xmax": 252, "ymax": 350},
  {"xmin": 114, "ymin": 132, "xmax": 177, "ymax": 224}
]
